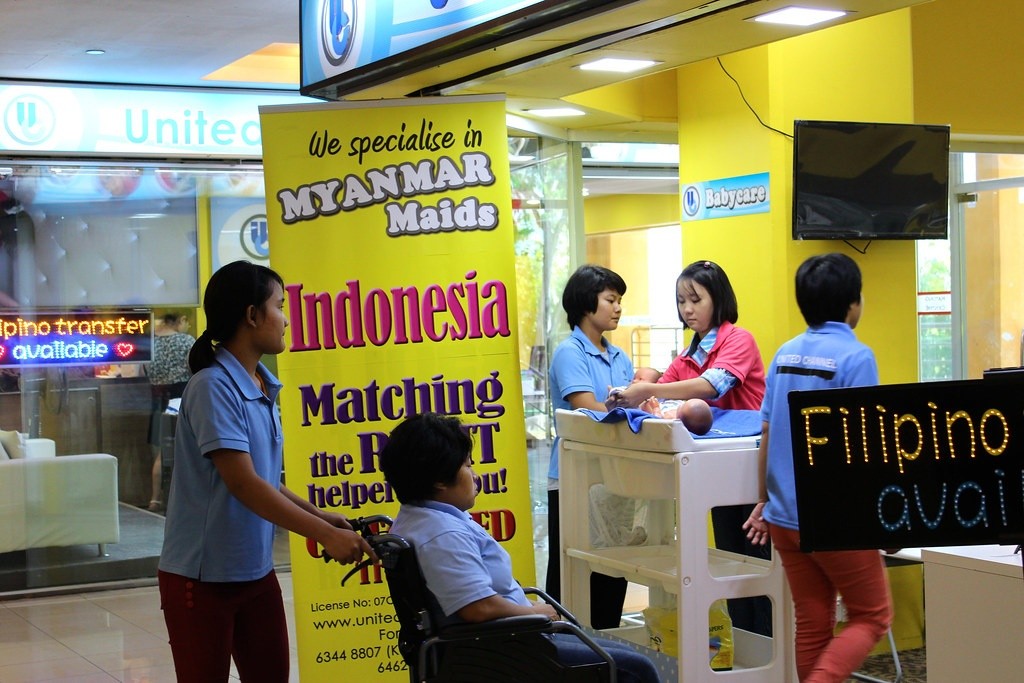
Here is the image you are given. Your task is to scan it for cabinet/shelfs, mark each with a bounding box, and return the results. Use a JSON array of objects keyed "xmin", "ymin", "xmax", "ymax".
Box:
[{"xmin": 555, "ymin": 408, "xmax": 786, "ymax": 683}]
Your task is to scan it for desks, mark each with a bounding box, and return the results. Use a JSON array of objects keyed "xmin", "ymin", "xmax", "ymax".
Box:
[{"xmin": 921, "ymin": 545, "xmax": 1024, "ymax": 683}]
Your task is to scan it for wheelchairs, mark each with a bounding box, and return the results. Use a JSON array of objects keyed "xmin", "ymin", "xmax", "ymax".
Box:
[{"xmin": 320, "ymin": 514, "xmax": 617, "ymax": 683}]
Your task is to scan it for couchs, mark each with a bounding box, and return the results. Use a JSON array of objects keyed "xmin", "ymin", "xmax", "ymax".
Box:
[{"xmin": 0, "ymin": 429, "xmax": 120, "ymax": 558}]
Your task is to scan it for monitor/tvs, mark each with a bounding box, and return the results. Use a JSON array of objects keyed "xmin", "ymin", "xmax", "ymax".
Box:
[{"xmin": 793, "ymin": 119, "xmax": 950, "ymax": 240}]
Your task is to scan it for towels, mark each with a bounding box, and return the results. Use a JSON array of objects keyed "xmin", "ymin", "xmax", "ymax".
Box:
[{"xmin": 577, "ymin": 398, "xmax": 763, "ymax": 440}]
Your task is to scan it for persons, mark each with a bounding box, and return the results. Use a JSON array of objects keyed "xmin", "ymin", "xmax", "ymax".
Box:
[
  {"xmin": 605, "ymin": 258, "xmax": 775, "ymax": 637},
  {"xmin": 144, "ymin": 308, "xmax": 195, "ymax": 514},
  {"xmin": 631, "ymin": 367, "xmax": 714, "ymax": 437},
  {"xmin": 379, "ymin": 414, "xmax": 660, "ymax": 683},
  {"xmin": 545, "ymin": 263, "xmax": 661, "ymax": 631},
  {"xmin": 743, "ymin": 251, "xmax": 900, "ymax": 683},
  {"xmin": 157, "ymin": 260, "xmax": 380, "ymax": 683}
]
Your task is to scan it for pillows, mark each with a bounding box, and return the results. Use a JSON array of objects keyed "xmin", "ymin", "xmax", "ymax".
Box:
[{"xmin": 0, "ymin": 429, "xmax": 27, "ymax": 459}]
